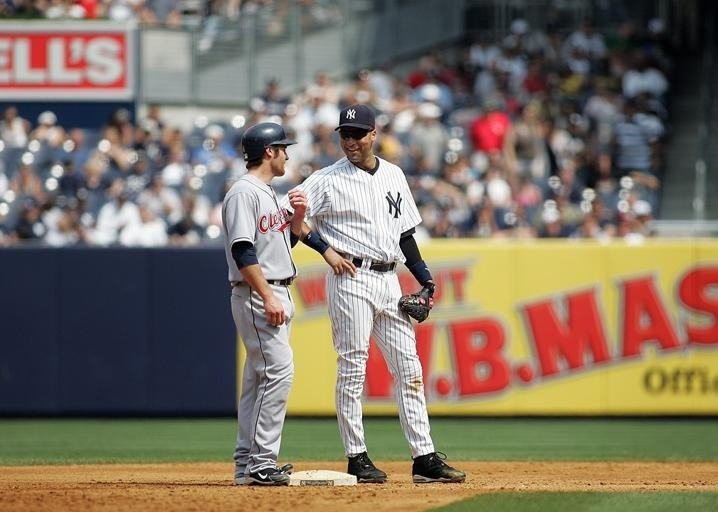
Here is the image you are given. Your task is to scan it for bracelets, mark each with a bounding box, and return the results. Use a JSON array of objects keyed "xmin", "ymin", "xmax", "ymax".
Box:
[
  {"xmin": 410, "ymin": 259, "xmax": 433, "ymax": 286},
  {"xmin": 300, "ymin": 229, "xmax": 331, "ymax": 256}
]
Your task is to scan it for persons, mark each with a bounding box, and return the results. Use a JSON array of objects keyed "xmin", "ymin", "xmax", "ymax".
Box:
[
  {"xmin": 220, "ymin": 121, "xmax": 310, "ymax": 486},
  {"xmin": 279, "ymin": 104, "xmax": 470, "ymax": 486},
  {"xmin": 0, "ymin": 1, "xmax": 718, "ymax": 249}
]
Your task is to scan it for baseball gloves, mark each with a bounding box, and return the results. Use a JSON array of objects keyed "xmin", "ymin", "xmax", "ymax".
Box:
[{"xmin": 399, "ymin": 282, "xmax": 434, "ymax": 323}]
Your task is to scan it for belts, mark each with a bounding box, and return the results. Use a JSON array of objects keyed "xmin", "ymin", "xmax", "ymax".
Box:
[
  {"xmin": 352, "ymin": 257, "xmax": 397, "ymax": 272},
  {"xmin": 231, "ymin": 275, "xmax": 295, "ymax": 287}
]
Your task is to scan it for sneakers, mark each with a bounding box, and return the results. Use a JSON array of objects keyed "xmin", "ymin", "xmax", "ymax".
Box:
[
  {"xmin": 412, "ymin": 452, "xmax": 467, "ymax": 485},
  {"xmin": 233, "ymin": 462, "xmax": 294, "ymax": 486},
  {"xmin": 347, "ymin": 451, "xmax": 388, "ymax": 484}
]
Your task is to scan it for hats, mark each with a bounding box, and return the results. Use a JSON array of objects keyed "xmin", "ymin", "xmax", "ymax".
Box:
[
  {"xmin": 241, "ymin": 122, "xmax": 299, "ymax": 161},
  {"xmin": 335, "ymin": 104, "xmax": 376, "ymax": 132}
]
